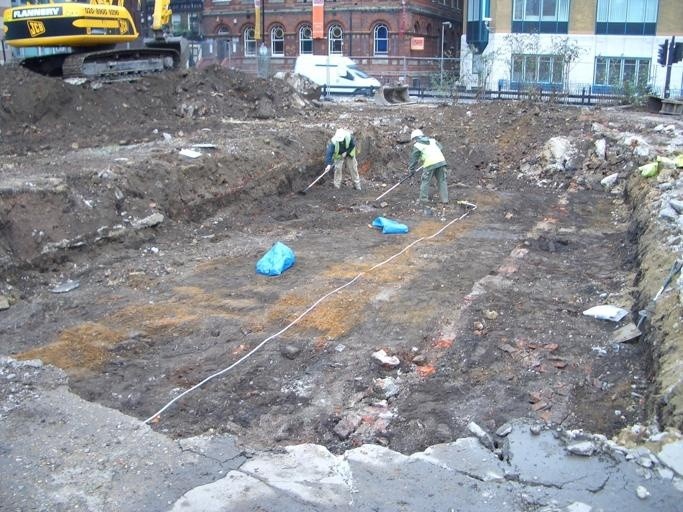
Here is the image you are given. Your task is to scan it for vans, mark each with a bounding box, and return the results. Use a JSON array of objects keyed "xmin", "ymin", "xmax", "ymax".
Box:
[{"xmin": 295, "ymin": 52, "xmax": 381, "ymax": 99}]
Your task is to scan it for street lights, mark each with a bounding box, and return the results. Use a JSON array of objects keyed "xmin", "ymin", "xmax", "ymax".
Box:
[{"xmin": 440, "ymin": 20, "xmax": 452, "ymax": 88}]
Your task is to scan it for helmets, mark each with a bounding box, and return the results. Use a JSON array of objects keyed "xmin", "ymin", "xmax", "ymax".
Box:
[
  {"xmin": 410, "ymin": 128, "xmax": 424, "ymax": 140},
  {"xmin": 334, "ymin": 128, "xmax": 346, "ymax": 142}
]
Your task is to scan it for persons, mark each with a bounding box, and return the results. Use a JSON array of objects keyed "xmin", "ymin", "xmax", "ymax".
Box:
[
  {"xmin": 324, "ymin": 128, "xmax": 362, "ymax": 191},
  {"xmin": 408, "ymin": 128, "xmax": 450, "ymax": 204}
]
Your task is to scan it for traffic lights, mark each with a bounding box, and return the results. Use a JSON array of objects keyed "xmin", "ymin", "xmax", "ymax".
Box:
[
  {"xmin": 674, "ymin": 43, "xmax": 683, "ymax": 62},
  {"xmin": 656, "ymin": 39, "xmax": 667, "ymax": 66}
]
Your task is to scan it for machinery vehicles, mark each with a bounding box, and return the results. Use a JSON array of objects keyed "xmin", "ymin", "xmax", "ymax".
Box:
[{"xmin": 0, "ymin": 0, "xmax": 183, "ymax": 84}]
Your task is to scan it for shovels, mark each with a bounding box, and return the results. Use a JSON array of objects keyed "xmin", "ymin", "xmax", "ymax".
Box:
[{"xmin": 612, "ymin": 259, "xmax": 683, "ymax": 342}]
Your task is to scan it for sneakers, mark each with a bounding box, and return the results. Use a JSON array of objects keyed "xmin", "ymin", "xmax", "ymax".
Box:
[
  {"xmin": 438, "ymin": 199, "xmax": 450, "ymax": 204},
  {"xmin": 334, "ymin": 184, "xmax": 362, "ymax": 191}
]
